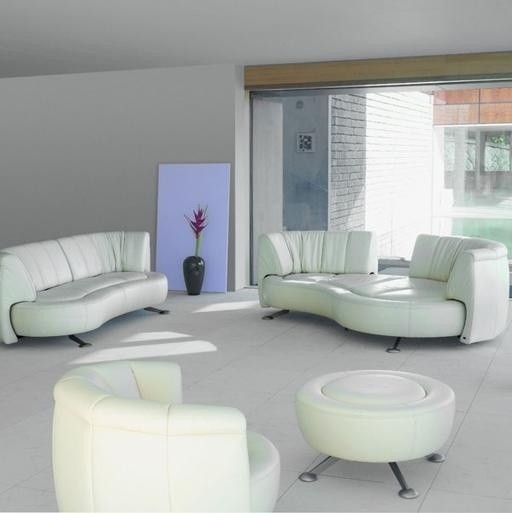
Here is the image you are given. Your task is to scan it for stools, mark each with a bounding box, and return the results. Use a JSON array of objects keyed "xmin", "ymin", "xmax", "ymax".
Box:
[{"xmin": 295, "ymin": 369, "xmax": 455, "ymax": 499}]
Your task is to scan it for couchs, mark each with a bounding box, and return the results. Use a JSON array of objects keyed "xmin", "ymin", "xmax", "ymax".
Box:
[
  {"xmin": 256, "ymin": 230, "xmax": 510, "ymax": 354},
  {"xmin": 0, "ymin": 230, "xmax": 170, "ymax": 348}
]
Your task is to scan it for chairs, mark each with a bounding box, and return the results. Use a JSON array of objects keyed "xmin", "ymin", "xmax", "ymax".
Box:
[{"xmin": 51, "ymin": 360, "xmax": 280, "ymax": 513}]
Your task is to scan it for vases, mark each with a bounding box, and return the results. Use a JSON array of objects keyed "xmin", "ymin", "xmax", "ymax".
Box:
[{"xmin": 183, "ymin": 256, "xmax": 205, "ymax": 295}]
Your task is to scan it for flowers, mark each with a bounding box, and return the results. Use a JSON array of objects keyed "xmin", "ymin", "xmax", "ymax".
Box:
[{"xmin": 184, "ymin": 203, "xmax": 208, "ymax": 258}]
